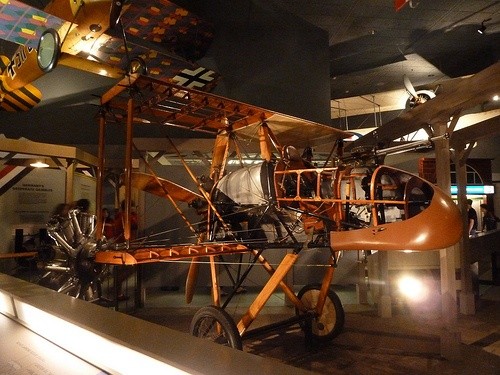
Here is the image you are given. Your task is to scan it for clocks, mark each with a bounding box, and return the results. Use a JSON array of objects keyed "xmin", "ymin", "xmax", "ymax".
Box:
[{"xmin": 37, "ymin": 28, "xmax": 61, "ymax": 74}]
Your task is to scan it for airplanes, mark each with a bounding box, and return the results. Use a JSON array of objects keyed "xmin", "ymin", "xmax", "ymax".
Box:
[{"xmin": 0, "ymin": 0, "xmax": 500, "ymax": 354}]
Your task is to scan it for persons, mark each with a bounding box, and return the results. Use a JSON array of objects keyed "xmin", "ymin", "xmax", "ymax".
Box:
[
  {"xmin": 480, "ymin": 203, "xmax": 497, "ymax": 232},
  {"xmin": 467, "ymin": 199, "xmax": 478, "ymax": 236},
  {"xmin": 101, "ymin": 199, "xmax": 136, "ymax": 301}
]
